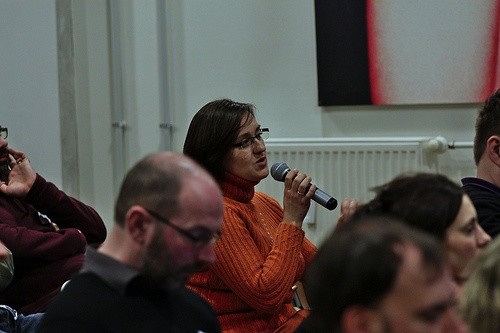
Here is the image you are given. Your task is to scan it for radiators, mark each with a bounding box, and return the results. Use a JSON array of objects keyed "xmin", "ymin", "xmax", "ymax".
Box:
[{"xmin": 255, "ymin": 135, "xmax": 448, "ymax": 247}]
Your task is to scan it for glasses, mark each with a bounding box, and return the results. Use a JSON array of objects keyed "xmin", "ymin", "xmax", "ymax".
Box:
[
  {"xmin": 0, "ymin": 128, "xmax": 8, "ymax": 139},
  {"xmin": 146, "ymin": 209, "xmax": 223, "ymax": 253},
  {"xmin": 234, "ymin": 127, "xmax": 270, "ymax": 154}
]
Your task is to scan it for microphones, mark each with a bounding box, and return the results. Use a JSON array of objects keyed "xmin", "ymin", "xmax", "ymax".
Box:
[{"xmin": 270, "ymin": 163, "xmax": 337, "ymax": 210}]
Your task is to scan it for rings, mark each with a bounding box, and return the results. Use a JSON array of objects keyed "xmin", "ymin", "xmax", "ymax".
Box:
[{"xmin": 297, "ymin": 192, "xmax": 304, "ymax": 195}]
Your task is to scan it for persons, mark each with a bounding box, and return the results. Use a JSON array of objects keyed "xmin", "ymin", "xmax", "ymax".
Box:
[
  {"xmin": 32, "ymin": 150, "xmax": 225, "ymax": 332},
  {"xmin": 183, "ymin": 99, "xmax": 359, "ymax": 333},
  {"xmin": 294, "ymin": 88, "xmax": 500, "ymax": 332},
  {"xmin": 0, "ymin": 125, "xmax": 107, "ymax": 333}
]
